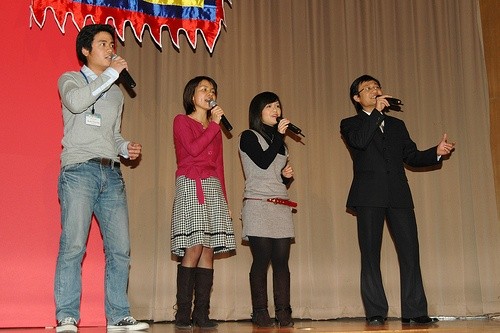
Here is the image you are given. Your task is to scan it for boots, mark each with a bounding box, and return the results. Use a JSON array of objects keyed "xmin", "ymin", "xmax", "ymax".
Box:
[
  {"xmin": 273, "ymin": 271, "xmax": 293, "ymax": 326},
  {"xmin": 191, "ymin": 265, "xmax": 219, "ymax": 327},
  {"xmin": 249, "ymin": 272, "xmax": 270, "ymax": 327},
  {"xmin": 172, "ymin": 264, "xmax": 196, "ymax": 327}
]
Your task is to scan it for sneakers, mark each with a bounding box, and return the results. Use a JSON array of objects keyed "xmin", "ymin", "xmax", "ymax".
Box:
[
  {"xmin": 55, "ymin": 315, "xmax": 78, "ymax": 332},
  {"xmin": 107, "ymin": 315, "xmax": 150, "ymax": 330}
]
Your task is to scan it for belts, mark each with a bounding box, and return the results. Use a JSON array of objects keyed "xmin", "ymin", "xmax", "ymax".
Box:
[
  {"xmin": 89, "ymin": 158, "xmax": 121, "ymax": 168},
  {"xmin": 246, "ymin": 198, "xmax": 298, "ymax": 207}
]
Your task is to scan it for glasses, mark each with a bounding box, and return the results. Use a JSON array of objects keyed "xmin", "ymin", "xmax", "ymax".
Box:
[{"xmin": 354, "ymin": 85, "xmax": 381, "ymax": 95}]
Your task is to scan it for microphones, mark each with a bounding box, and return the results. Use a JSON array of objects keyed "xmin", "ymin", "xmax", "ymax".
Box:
[
  {"xmin": 112, "ymin": 55, "xmax": 136, "ymax": 89},
  {"xmin": 210, "ymin": 101, "xmax": 232, "ymax": 131},
  {"xmin": 376, "ymin": 95, "xmax": 404, "ymax": 105},
  {"xmin": 276, "ymin": 117, "xmax": 305, "ymax": 137}
]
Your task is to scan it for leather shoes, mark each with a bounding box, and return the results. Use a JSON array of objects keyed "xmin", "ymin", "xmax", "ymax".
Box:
[
  {"xmin": 369, "ymin": 316, "xmax": 383, "ymax": 325},
  {"xmin": 401, "ymin": 316, "xmax": 439, "ymax": 323}
]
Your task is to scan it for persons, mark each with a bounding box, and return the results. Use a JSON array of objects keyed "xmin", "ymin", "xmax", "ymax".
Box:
[
  {"xmin": 170, "ymin": 76, "xmax": 237, "ymax": 329},
  {"xmin": 340, "ymin": 75, "xmax": 458, "ymax": 326},
  {"xmin": 236, "ymin": 92, "xmax": 297, "ymax": 327},
  {"xmin": 55, "ymin": 24, "xmax": 150, "ymax": 333}
]
